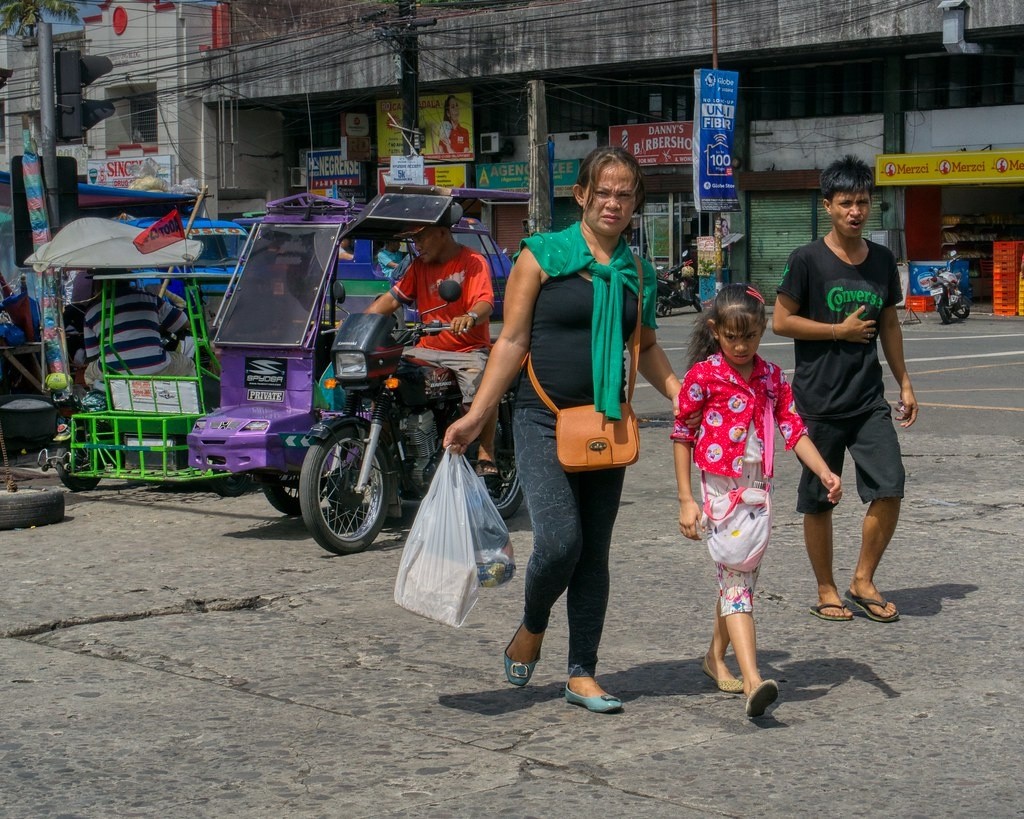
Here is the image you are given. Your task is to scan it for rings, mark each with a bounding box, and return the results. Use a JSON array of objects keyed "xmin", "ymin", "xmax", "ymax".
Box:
[{"xmin": 868, "ymin": 334, "xmax": 869, "ymax": 338}]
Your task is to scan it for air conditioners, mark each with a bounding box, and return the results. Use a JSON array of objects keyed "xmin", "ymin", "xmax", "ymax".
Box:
[
  {"xmin": 480, "ymin": 132, "xmax": 505, "ymax": 154},
  {"xmin": 291, "ymin": 167, "xmax": 307, "ymax": 188}
]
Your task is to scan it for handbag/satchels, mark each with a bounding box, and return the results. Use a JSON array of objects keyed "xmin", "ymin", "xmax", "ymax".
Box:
[
  {"xmin": 393, "ymin": 444, "xmax": 516, "ymax": 629},
  {"xmin": 700, "ymin": 486, "xmax": 770, "ymax": 571},
  {"xmin": 555, "ymin": 401, "xmax": 640, "ymax": 473}
]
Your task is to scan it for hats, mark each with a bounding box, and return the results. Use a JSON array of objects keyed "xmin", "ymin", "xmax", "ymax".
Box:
[{"xmin": 393, "ymin": 216, "xmax": 451, "ymax": 240}]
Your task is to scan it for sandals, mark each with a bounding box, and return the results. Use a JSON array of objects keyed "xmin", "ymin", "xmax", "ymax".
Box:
[{"xmin": 475, "ymin": 459, "xmax": 497, "ymax": 483}]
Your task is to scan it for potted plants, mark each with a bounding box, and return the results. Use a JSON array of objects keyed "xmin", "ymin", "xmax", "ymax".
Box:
[{"xmin": 697, "ymin": 258, "xmax": 716, "ymax": 313}]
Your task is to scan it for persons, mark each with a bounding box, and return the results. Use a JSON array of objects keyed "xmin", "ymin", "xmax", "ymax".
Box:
[
  {"xmin": 83, "ymin": 267, "xmax": 197, "ymax": 388},
  {"xmin": 442, "ymin": 145, "xmax": 682, "ymax": 713},
  {"xmin": 771, "ymin": 153, "xmax": 919, "ymax": 623},
  {"xmin": 430, "ymin": 95, "xmax": 470, "ymax": 154},
  {"xmin": 336, "ymin": 222, "xmax": 499, "ymax": 476},
  {"xmin": 669, "ymin": 285, "xmax": 843, "ymax": 719},
  {"xmin": 377, "ymin": 239, "xmax": 413, "ymax": 278},
  {"xmin": 63, "ymin": 269, "xmax": 94, "ymax": 329},
  {"xmin": 339, "ymin": 238, "xmax": 353, "ymax": 260},
  {"xmin": 721, "ymin": 219, "xmax": 728, "ymax": 236}
]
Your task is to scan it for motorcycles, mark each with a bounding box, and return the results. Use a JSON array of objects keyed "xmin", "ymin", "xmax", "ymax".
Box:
[
  {"xmin": 187, "ymin": 190, "xmax": 523, "ymax": 555},
  {"xmin": 656, "ymin": 250, "xmax": 702, "ymax": 317},
  {"xmin": 928, "ymin": 250, "xmax": 972, "ymax": 325},
  {"xmin": 36, "ymin": 374, "xmax": 114, "ymax": 491}
]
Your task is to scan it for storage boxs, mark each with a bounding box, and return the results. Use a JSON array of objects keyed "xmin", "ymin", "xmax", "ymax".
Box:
[
  {"xmin": 993, "ymin": 241, "xmax": 1024, "ymax": 316},
  {"xmin": 906, "ymin": 296, "xmax": 935, "ymax": 312}
]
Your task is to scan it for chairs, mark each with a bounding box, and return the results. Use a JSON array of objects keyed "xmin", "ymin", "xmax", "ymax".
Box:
[{"xmin": 979, "ymin": 260, "xmax": 993, "ymax": 303}]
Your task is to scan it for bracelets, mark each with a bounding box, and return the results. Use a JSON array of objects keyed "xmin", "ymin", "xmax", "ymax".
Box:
[{"xmin": 832, "ymin": 324, "xmax": 836, "ymax": 340}]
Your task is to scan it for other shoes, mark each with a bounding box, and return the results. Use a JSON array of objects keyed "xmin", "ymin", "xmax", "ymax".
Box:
[
  {"xmin": 503, "ymin": 621, "xmax": 542, "ymax": 686},
  {"xmin": 702, "ymin": 654, "xmax": 745, "ymax": 693},
  {"xmin": 746, "ymin": 680, "xmax": 778, "ymax": 717},
  {"xmin": 565, "ymin": 681, "xmax": 622, "ymax": 712}
]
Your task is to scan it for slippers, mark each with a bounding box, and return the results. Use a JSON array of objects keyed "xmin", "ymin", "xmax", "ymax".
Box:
[
  {"xmin": 810, "ymin": 604, "xmax": 852, "ymax": 621},
  {"xmin": 845, "ymin": 590, "xmax": 900, "ymax": 621}
]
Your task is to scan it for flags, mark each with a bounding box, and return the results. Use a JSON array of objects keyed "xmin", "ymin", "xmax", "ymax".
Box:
[{"xmin": 133, "ymin": 210, "xmax": 185, "ymax": 255}]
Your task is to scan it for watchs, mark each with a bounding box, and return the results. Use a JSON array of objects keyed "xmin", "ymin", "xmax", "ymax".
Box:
[{"xmin": 466, "ymin": 312, "xmax": 478, "ymax": 326}]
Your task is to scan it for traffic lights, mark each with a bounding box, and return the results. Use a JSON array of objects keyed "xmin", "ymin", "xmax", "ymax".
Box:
[{"xmin": 56, "ymin": 51, "xmax": 116, "ymax": 140}]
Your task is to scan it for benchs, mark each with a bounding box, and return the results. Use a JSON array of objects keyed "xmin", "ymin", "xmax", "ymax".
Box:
[{"xmin": 105, "ymin": 373, "xmax": 207, "ymax": 415}]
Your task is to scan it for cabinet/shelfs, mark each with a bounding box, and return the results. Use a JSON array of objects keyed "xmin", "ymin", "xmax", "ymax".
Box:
[{"xmin": 940, "ymin": 210, "xmax": 1024, "ymax": 297}]
